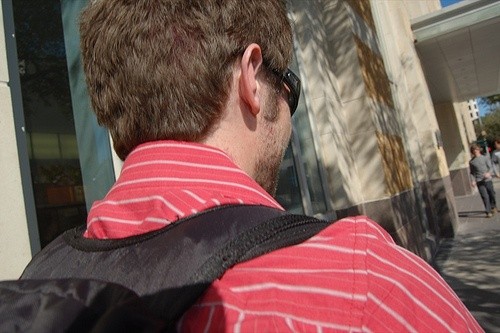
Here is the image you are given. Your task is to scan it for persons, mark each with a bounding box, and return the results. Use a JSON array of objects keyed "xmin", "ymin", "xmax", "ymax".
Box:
[
  {"xmin": 469, "ymin": 129, "xmax": 500, "ymax": 218},
  {"xmin": 17, "ymin": 0, "xmax": 488, "ymax": 333}
]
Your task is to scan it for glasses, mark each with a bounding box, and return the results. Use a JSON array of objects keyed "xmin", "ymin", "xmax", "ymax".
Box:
[{"xmin": 253, "ymin": 50, "xmax": 301, "ymax": 116}]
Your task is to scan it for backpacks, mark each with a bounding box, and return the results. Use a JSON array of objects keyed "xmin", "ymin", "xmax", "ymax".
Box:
[{"xmin": 0, "ymin": 203, "xmax": 334, "ymax": 332}]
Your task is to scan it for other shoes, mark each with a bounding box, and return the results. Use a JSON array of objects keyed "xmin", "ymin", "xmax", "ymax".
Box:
[
  {"xmin": 487, "ymin": 211, "xmax": 492, "ymax": 217},
  {"xmin": 492, "ymin": 207, "xmax": 497, "ymax": 213}
]
